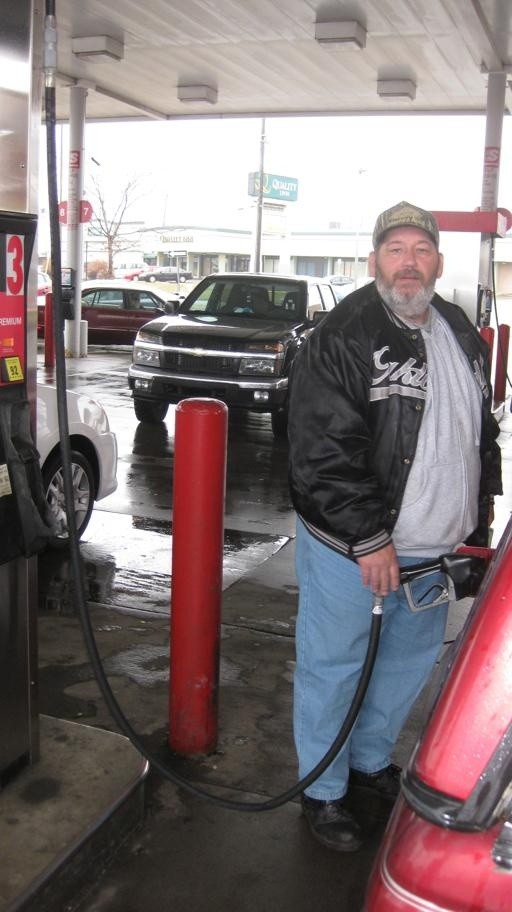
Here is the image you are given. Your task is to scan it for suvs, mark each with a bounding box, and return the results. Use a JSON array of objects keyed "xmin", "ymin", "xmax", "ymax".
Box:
[{"xmin": 127, "ymin": 273, "xmax": 340, "ymax": 438}]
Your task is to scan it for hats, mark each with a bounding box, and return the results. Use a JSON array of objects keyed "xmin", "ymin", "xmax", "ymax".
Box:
[{"xmin": 372, "ymin": 201, "xmax": 440, "ymax": 252}]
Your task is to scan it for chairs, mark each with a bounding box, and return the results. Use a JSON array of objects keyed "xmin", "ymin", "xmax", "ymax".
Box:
[{"xmin": 216, "ymin": 283, "xmax": 299, "ymax": 319}]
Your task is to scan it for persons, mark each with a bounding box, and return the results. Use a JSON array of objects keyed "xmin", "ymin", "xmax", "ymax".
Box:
[{"xmin": 287, "ymin": 201, "xmax": 503, "ymax": 853}]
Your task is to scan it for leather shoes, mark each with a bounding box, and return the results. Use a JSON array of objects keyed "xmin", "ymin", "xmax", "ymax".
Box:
[
  {"xmin": 349, "ymin": 763, "xmax": 402, "ymax": 801},
  {"xmin": 301, "ymin": 791, "xmax": 365, "ymax": 852}
]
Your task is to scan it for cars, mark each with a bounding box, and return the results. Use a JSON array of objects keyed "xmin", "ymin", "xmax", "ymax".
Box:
[
  {"xmin": 36, "ymin": 271, "xmax": 226, "ymax": 345},
  {"xmin": 35, "ymin": 382, "xmax": 119, "ymax": 547},
  {"xmin": 123, "ymin": 265, "xmax": 193, "ymax": 283}
]
